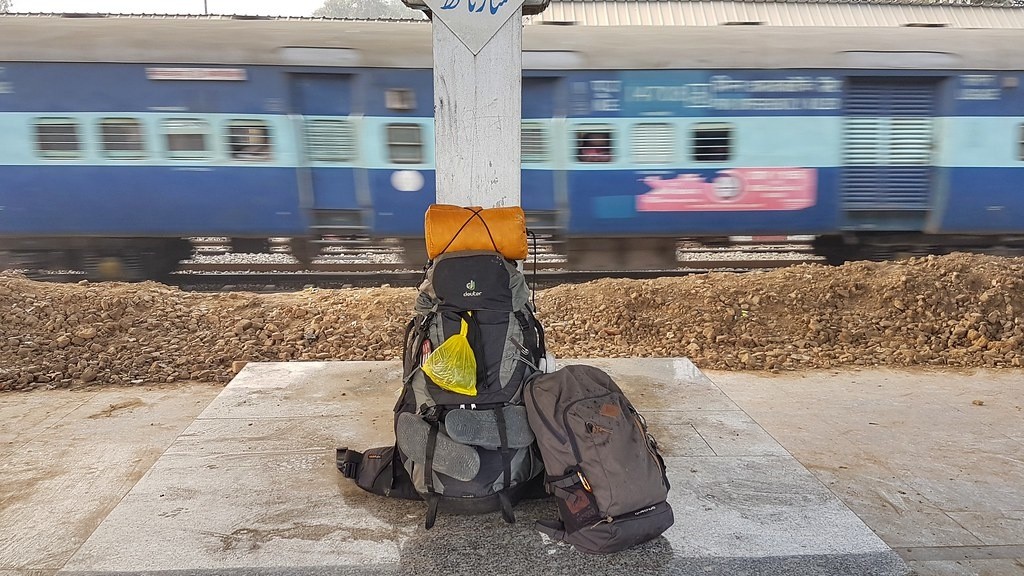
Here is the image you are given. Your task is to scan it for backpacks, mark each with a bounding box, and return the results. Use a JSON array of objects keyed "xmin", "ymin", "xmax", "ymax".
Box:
[
  {"xmin": 337, "ymin": 203, "xmax": 557, "ymax": 529},
  {"xmin": 521, "ymin": 365, "xmax": 675, "ymax": 554}
]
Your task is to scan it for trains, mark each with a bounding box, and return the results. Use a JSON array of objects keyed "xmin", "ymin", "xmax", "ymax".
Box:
[{"xmin": 0, "ymin": 10, "xmax": 1023, "ymax": 282}]
